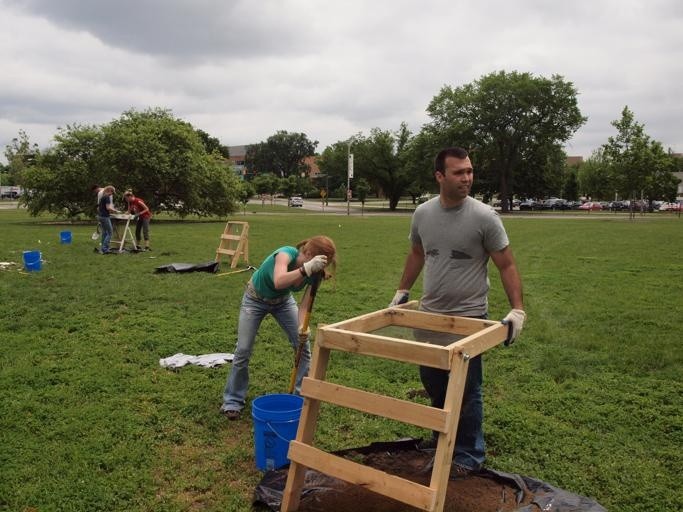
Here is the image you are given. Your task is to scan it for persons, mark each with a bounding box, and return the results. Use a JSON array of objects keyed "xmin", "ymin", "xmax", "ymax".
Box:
[
  {"xmin": 385, "ymin": 145, "xmax": 527, "ymax": 481},
  {"xmin": 220, "ymin": 235, "xmax": 338, "ymax": 421},
  {"xmin": 120, "ymin": 187, "xmax": 152, "ymax": 251},
  {"xmin": 96, "ymin": 185, "xmax": 118, "ymax": 254},
  {"xmin": 91, "ymin": 183, "xmax": 114, "ymax": 208}
]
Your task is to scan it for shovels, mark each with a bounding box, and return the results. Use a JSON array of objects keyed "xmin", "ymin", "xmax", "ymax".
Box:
[{"xmin": 217, "ymin": 265, "xmax": 258, "ymax": 277}]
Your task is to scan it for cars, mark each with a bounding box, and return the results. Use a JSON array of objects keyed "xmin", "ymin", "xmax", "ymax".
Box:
[
  {"xmin": 0, "ymin": 187, "xmax": 38, "ymax": 201},
  {"xmin": 288, "ymin": 196, "xmax": 304, "ymax": 207},
  {"xmin": 493, "ymin": 196, "xmax": 683, "ymax": 214}
]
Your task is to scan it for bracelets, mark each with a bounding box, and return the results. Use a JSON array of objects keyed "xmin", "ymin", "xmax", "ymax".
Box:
[{"xmin": 298, "ymin": 266, "xmax": 307, "ymax": 278}]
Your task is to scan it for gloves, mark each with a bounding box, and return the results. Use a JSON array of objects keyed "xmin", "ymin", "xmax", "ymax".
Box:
[
  {"xmin": 296, "ymin": 325, "xmax": 310, "ymax": 345},
  {"xmin": 387, "ymin": 289, "xmax": 410, "ymax": 311},
  {"xmin": 499, "ymin": 309, "xmax": 526, "ymax": 346},
  {"xmin": 303, "ymin": 255, "xmax": 328, "ymax": 279}
]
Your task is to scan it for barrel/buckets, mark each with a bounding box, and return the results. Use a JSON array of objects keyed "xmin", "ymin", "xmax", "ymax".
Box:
[
  {"xmin": 252, "ymin": 394, "xmax": 304, "ymax": 470},
  {"xmin": 61, "ymin": 232, "xmax": 71, "ymax": 244},
  {"xmin": 23, "ymin": 251, "xmax": 41, "ymax": 271}
]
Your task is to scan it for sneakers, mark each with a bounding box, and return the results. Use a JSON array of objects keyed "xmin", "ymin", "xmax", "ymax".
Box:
[
  {"xmin": 422, "ymin": 452, "xmax": 437, "ymax": 475},
  {"xmin": 100, "ymin": 246, "xmax": 151, "ymax": 255},
  {"xmin": 432, "ymin": 465, "xmax": 474, "ymax": 479},
  {"xmin": 226, "ymin": 410, "xmax": 239, "ymax": 420}
]
